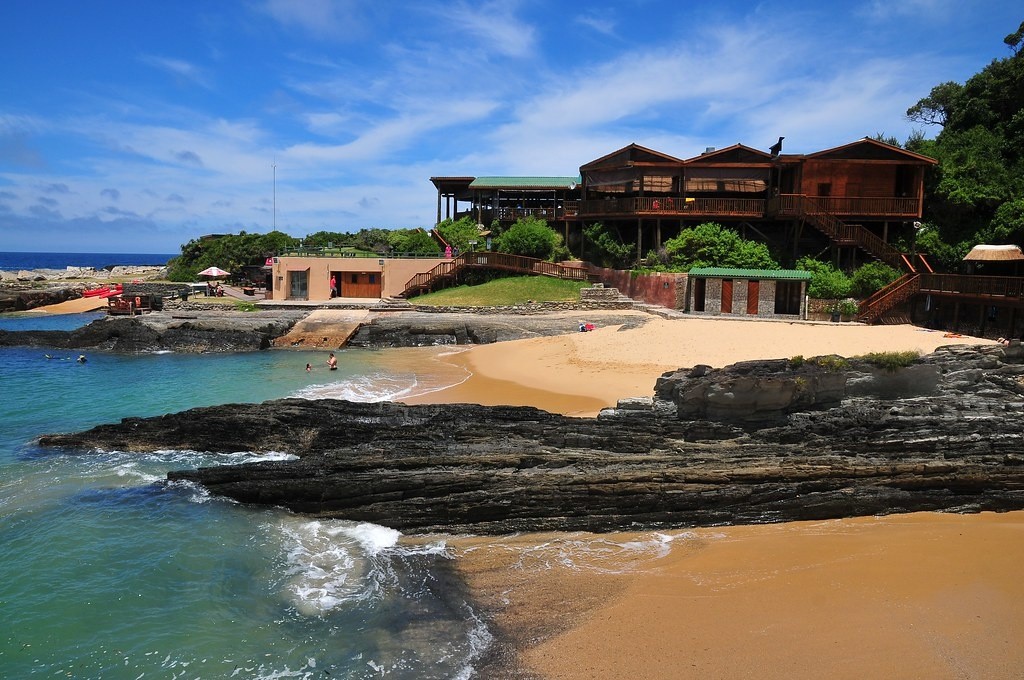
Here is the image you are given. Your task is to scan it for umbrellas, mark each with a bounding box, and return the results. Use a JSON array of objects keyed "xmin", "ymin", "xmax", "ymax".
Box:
[
  {"xmin": 196, "ymin": 266, "xmax": 232, "ymax": 287},
  {"xmin": 962, "ymin": 245, "xmax": 1024, "ymax": 262}
]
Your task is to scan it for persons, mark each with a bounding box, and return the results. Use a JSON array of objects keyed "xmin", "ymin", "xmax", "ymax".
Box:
[
  {"xmin": 445, "ymin": 244, "xmax": 460, "ymax": 258},
  {"xmin": 330, "ymin": 275, "xmax": 338, "ymax": 299},
  {"xmin": 306, "ymin": 363, "xmax": 312, "ymax": 372},
  {"xmin": 326, "ymin": 353, "xmax": 338, "ymax": 370},
  {"xmin": 207, "ymin": 282, "xmax": 224, "ymax": 297}
]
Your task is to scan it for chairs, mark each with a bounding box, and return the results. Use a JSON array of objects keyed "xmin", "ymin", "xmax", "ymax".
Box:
[{"xmin": 684, "ymin": 197, "xmax": 695, "ymax": 210}]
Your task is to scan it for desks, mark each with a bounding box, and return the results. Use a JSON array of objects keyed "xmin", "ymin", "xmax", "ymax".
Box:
[{"xmin": 209, "ymin": 286, "xmax": 223, "ymax": 297}]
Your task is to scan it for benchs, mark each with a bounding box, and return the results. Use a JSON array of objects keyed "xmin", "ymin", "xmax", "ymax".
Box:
[
  {"xmin": 101, "ymin": 307, "xmax": 152, "ymax": 316},
  {"xmin": 241, "ymin": 287, "xmax": 255, "ymax": 296},
  {"xmin": 204, "ymin": 290, "xmax": 226, "ymax": 297}
]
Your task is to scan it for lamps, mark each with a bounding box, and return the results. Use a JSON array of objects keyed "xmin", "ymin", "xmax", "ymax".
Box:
[{"xmin": 664, "ymin": 282, "xmax": 668, "ymax": 289}]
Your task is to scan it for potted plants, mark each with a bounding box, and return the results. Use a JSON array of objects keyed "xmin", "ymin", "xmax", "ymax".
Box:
[{"xmin": 825, "ymin": 299, "xmax": 858, "ymax": 322}]
